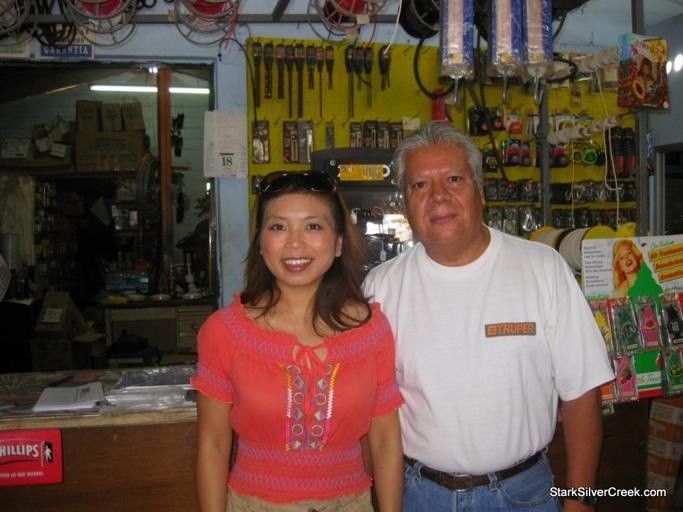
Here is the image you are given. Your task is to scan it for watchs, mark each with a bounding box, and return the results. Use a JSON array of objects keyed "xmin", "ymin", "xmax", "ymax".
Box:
[{"xmin": 564, "ymin": 488, "xmax": 597, "ymax": 507}]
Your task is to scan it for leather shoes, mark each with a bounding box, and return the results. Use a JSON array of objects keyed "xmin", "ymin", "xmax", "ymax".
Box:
[{"xmin": 403, "ymin": 449, "xmax": 543, "ymax": 492}]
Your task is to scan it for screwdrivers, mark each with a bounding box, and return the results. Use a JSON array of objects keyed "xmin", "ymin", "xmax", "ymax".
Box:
[
  {"xmin": 364, "ymin": 49, "xmax": 372, "ymax": 108},
  {"xmin": 286, "ymin": 46, "xmax": 293, "ymax": 119},
  {"xmin": 317, "ymin": 47, "xmax": 324, "ymax": 118}
]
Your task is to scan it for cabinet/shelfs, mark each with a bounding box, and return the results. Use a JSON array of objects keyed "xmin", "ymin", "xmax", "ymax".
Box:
[{"xmin": 0, "ymin": 366, "xmax": 198, "ymax": 512}]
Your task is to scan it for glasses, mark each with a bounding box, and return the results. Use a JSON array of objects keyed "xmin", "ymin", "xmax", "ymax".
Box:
[{"xmin": 258, "ymin": 170, "xmax": 337, "ymax": 192}]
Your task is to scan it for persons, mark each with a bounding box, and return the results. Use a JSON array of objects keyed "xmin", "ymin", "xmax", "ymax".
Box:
[
  {"xmin": 608, "ymin": 239, "xmax": 643, "ymax": 300},
  {"xmin": 189, "ymin": 170, "xmax": 405, "ymax": 512},
  {"xmin": 359, "ymin": 120, "xmax": 616, "ymax": 512}
]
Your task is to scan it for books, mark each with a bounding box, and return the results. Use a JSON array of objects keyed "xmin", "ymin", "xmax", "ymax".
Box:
[{"xmin": 32, "ymin": 366, "xmax": 196, "ymax": 413}]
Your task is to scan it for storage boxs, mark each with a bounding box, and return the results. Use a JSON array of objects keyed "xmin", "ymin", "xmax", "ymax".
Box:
[
  {"xmin": 2, "ymin": 101, "xmax": 146, "ymax": 173},
  {"xmin": 27, "ymin": 291, "xmax": 104, "ymax": 371},
  {"xmin": 173, "ymin": 305, "xmax": 216, "ymax": 354}
]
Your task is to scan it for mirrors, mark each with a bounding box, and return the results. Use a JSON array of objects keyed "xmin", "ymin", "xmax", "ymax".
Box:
[{"xmin": 0, "ymin": 54, "xmax": 216, "ymax": 310}]
